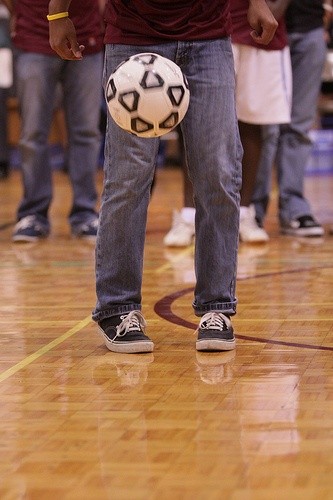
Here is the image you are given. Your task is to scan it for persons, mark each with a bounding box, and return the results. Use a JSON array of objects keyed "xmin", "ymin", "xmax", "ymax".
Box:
[
  {"xmin": 164, "ymin": 0, "xmax": 293, "ymax": 248},
  {"xmin": 48, "ymin": 0, "xmax": 278, "ymax": 353},
  {"xmin": 0, "ymin": 0, "xmax": 106, "ymax": 241},
  {"xmin": 251, "ymin": 0, "xmax": 324, "ymax": 238},
  {"xmin": 307, "ymin": 0, "xmax": 333, "ymax": 147}
]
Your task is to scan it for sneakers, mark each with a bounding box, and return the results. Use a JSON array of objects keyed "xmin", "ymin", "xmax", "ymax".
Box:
[
  {"xmin": 96, "ymin": 311, "xmax": 155, "ymax": 353},
  {"xmin": 94, "ymin": 351, "xmax": 154, "ymax": 394},
  {"xmin": 237, "ymin": 241, "xmax": 269, "ymax": 278},
  {"xmin": 163, "ymin": 209, "xmax": 196, "ymax": 247},
  {"xmin": 280, "ymin": 216, "xmax": 324, "ymax": 236},
  {"xmin": 11, "ymin": 216, "xmax": 50, "ymax": 242},
  {"xmin": 194, "ymin": 312, "xmax": 236, "ymax": 351},
  {"xmin": 195, "ymin": 349, "xmax": 235, "ymax": 386},
  {"xmin": 238, "ymin": 203, "xmax": 270, "ymax": 242},
  {"xmin": 70, "ymin": 216, "xmax": 99, "ymax": 240},
  {"xmin": 164, "ymin": 246, "xmax": 196, "ymax": 284}
]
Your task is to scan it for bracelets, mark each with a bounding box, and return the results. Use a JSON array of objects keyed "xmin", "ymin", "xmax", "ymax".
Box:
[{"xmin": 47, "ymin": 12, "xmax": 69, "ymax": 21}]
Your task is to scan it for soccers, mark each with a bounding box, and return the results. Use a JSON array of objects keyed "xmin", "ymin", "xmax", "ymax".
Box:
[{"xmin": 105, "ymin": 52, "xmax": 191, "ymax": 139}]
